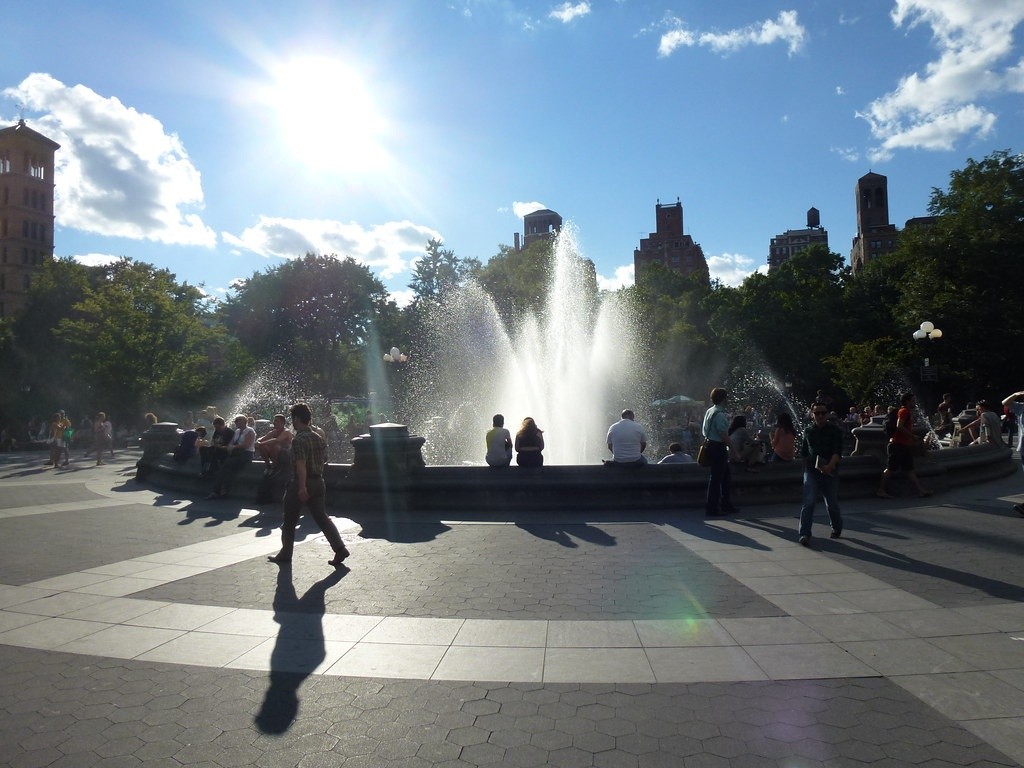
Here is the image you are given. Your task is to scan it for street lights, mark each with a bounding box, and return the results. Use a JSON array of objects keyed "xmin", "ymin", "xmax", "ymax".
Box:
[
  {"xmin": 382, "ymin": 346, "xmax": 408, "ymax": 423},
  {"xmin": 913, "ymin": 321, "xmax": 944, "ymax": 371}
]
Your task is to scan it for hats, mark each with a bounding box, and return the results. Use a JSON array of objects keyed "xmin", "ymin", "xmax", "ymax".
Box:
[{"xmin": 943, "ymin": 393, "xmax": 952, "ymax": 399}]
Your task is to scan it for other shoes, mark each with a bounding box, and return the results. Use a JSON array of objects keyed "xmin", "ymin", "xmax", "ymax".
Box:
[
  {"xmin": 44, "ymin": 461, "xmax": 53, "ymax": 465},
  {"xmin": 876, "ymin": 490, "xmax": 895, "ymax": 499},
  {"xmin": 918, "ymin": 491, "xmax": 934, "ymax": 499},
  {"xmin": 268, "ymin": 468, "xmax": 280, "ymax": 479},
  {"xmin": 704, "ymin": 510, "xmax": 725, "ymax": 520},
  {"xmin": 799, "ymin": 535, "xmax": 807, "ymax": 545},
  {"xmin": 267, "ymin": 552, "xmax": 292, "ymax": 562},
  {"xmin": 1013, "ymin": 504, "xmax": 1024, "ymax": 516},
  {"xmin": 328, "ymin": 549, "xmax": 350, "ymax": 565},
  {"xmin": 264, "ymin": 465, "xmax": 271, "ymax": 475},
  {"xmin": 723, "ymin": 506, "xmax": 741, "ymax": 514},
  {"xmin": 55, "ymin": 464, "xmax": 58, "ymax": 467},
  {"xmin": 96, "ymin": 461, "xmax": 105, "ymax": 465},
  {"xmin": 220, "ymin": 488, "xmax": 231, "ymax": 496},
  {"xmin": 830, "ymin": 530, "xmax": 839, "ymax": 539},
  {"xmin": 204, "ymin": 491, "xmax": 218, "ymax": 500},
  {"xmin": 62, "ymin": 462, "xmax": 69, "ymax": 466}
]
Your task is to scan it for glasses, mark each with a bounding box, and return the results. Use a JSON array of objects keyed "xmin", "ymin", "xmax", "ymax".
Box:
[{"xmin": 813, "ymin": 411, "xmax": 827, "ymax": 416}]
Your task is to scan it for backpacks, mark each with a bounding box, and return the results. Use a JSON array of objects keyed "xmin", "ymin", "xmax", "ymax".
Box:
[{"xmin": 883, "ymin": 407, "xmax": 909, "ymax": 438}]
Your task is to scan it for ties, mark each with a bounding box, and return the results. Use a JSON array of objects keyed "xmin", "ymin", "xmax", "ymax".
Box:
[{"xmin": 235, "ymin": 430, "xmax": 242, "ymax": 444}]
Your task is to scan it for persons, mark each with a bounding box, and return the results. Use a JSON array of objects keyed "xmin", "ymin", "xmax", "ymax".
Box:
[
  {"xmin": 144, "ymin": 405, "xmax": 327, "ymax": 501},
  {"xmin": 0, "ymin": 407, "xmax": 138, "ymax": 469},
  {"xmin": 729, "ymin": 415, "xmax": 763, "ymax": 473},
  {"xmin": 606, "ymin": 408, "xmax": 648, "ymax": 465},
  {"xmin": 799, "ymin": 399, "xmax": 844, "ymax": 545},
  {"xmin": 515, "ymin": 417, "xmax": 544, "ymax": 466},
  {"xmin": 656, "ymin": 443, "xmax": 694, "ymax": 464},
  {"xmin": 485, "ymin": 414, "xmax": 512, "ymax": 466},
  {"xmin": 769, "ymin": 412, "xmax": 799, "ymax": 462},
  {"xmin": 701, "ymin": 387, "xmax": 742, "ymax": 517},
  {"xmin": 846, "ymin": 393, "xmax": 1018, "ymax": 447},
  {"xmin": 874, "ymin": 392, "xmax": 936, "ymax": 499},
  {"xmin": 267, "ymin": 403, "xmax": 350, "ymax": 565}
]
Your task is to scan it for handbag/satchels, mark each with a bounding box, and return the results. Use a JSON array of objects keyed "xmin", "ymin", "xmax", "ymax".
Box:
[
  {"xmin": 62, "ymin": 428, "xmax": 72, "ymax": 441},
  {"xmin": 46, "ymin": 437, "xmax": 54, "ymax": 444},
  {"xmin": 745, "ymin": 442, "xmax": 766, "ymax": 467},
  {"xmin": 697, "ymin": 445, "xmax": 711, "ymax": 466}
]
